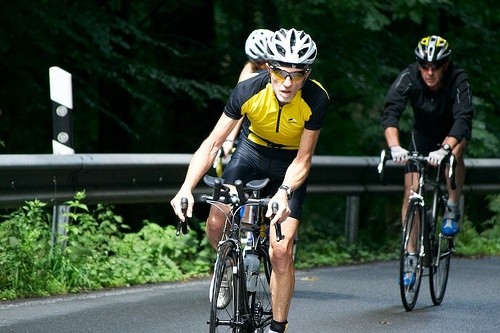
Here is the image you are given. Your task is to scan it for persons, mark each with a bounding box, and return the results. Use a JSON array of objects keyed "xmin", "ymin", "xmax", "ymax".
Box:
[
  {"xmin": 171, "ymin": 27, "xmax": 330, "ymax": 333},
  {"xmin": 217, "ymin": 29, "xmax": 302, "ymax": 293},
  {"xmin": 383, "ymin": 36, "xmax": 473, "ymax": 288}
]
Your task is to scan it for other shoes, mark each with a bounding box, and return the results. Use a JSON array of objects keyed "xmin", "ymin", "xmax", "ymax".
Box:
[
  {"xmin": 441, "ymin": 205, "xmax": 461, "ymax": 235},
  {"xmin": 397, "ymin": 251, "xmax": 419, "ymax": 289},
  {"xmin": 208, "ymin": 251, "xmax": 233, "ymax": 309}
]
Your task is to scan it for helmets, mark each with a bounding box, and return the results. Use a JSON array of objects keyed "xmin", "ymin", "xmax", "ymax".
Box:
[
  {"xmin": 244, "ymin": 29, "xmax": 275, "ymax": 62},
  {"xmin": 264, "ymin": 28, "xmax": 318, "ymax": 65},
  {"xmin": 414, "ymin": 36, "xmax": 452, "ymax": 62}
]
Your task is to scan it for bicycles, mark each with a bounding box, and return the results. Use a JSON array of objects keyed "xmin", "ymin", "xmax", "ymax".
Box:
[
  {"xmin": 377, "ymin": 149, "xmax": 457, "ymax": 312},
  {"xmin": 175, "ymin": 176, "xmax": 299, "ymax": 333}
]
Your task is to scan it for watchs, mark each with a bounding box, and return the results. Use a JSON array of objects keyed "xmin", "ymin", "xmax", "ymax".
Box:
[
  {"xmin": 441, "ymin": 144, "xmax": 452, "ymax": 152},
  {"xmin": 278, "ymin": 185, "xmax": 294, "ymax": 201}
]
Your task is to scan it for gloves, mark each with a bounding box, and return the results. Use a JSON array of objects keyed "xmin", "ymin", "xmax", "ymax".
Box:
[
  {"xmin": 427, "ymin": 149, "xmax": 448, "ymax": 167},
  {"xmin": 389, "ymin": 145, "xmax": 411, "ymax": 165}
]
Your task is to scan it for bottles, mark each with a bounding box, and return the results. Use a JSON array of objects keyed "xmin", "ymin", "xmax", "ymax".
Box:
[
  {"xmin": 243, "ymin": 250, "xmax": 260, "ymax": 293},
  {"xmin": 426, "ymin": 206, "xmax": 435, "ymax": 236}
]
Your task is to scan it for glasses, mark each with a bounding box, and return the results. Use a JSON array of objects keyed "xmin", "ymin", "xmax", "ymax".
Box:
[
  {"xmin": 418, "ymin": 60, "xmax": 447, "ymax": 70},
  {"xmin": 268, "ymin": 63, "xmax": 309, "ymax": 83}
]
